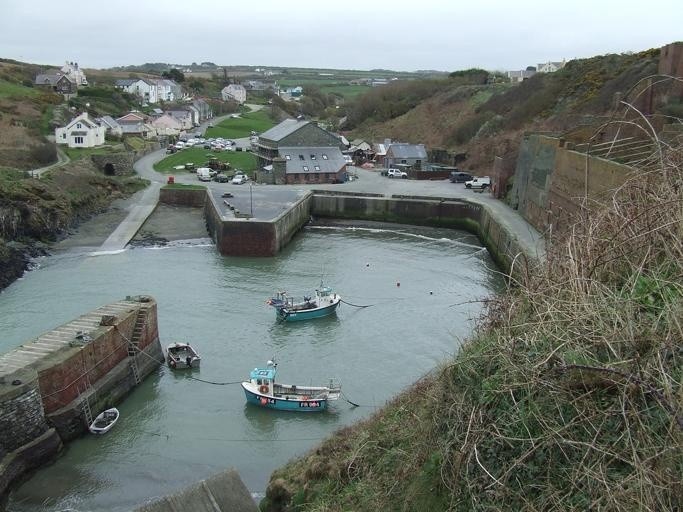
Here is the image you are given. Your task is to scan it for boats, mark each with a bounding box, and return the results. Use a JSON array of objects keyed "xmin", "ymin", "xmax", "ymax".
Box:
[
  {"xmin": 240, "ymin": 354, "xmax": 344, "ymax": 413},
  {"xmin": 164, "ymin": 341, "xmax": 202, "ymax": 370},
  {"xmin": 265, "ymin": 263, "xmax": 344, "ymax": 326},
  {"xmin": 87, "ymin": 406, "xmax": 120, "ymax": 437}
]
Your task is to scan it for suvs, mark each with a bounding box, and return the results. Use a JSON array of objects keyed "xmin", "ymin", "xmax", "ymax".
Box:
[
  {"xmin": 463, "ymin": 175, "xmax": 492, "ymax": 190},
  {"xmin": 387, "ymin": 168, "xmax": 408, "ymax": 179}
]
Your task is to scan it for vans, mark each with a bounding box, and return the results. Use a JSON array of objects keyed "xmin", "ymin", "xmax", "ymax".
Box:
[{"xmin": 448, "ymin": 171, "xmax": 473, "ymax": 185}]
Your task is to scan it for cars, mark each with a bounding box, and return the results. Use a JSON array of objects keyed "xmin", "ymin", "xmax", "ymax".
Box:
[
  {"xmin": 220, "ymin": 191, "xmax": 233, "ymax": 197},
  {"xmin": 162, "ymin": 113, "xmax": 250, "ymax": 187},
  {"xmin": 343, "ymin": 155, "xmax": 353, "ymax": 165}
]
